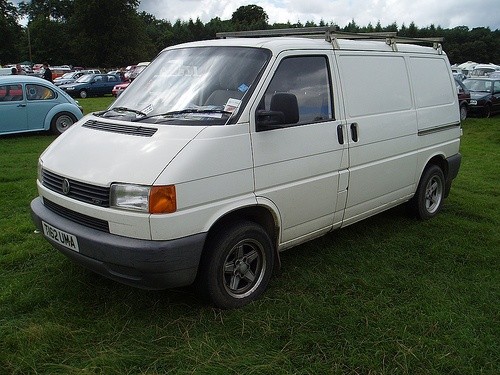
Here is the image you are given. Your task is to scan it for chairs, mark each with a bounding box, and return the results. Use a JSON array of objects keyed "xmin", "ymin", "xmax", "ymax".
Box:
[
  {"xmin": 97, "ymin": 79, "xmax": 102, "ymax": 83},
  {"xmin": 205, "ymin": 64, "xmax": 248, "ymax": 106}
]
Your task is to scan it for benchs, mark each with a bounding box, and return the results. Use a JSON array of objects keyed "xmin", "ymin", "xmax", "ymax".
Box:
[{"xmin": 108, "ymin": 78, "xmax": 116, "ymax": 82}]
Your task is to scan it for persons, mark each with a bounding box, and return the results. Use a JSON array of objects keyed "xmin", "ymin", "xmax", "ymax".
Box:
[
  {"xmin": 16, "ymin": 64, "xmax": 27, "ymax": 74},
  {"xmin": 43, "ymin": 62, "xmax": 52, "ymax": 82},
  {"xmin": 12, "ymin": 68, "xmax": 17, "ymax": 74}
]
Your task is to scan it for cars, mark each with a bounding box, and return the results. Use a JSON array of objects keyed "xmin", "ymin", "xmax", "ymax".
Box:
[
  {"xmin": 0, "ymin": 60, "xmax": 151, "ymax": 99},
  {"xmin": 450, "ymin": 61, "xmax": 500, "ymax": 122},
  {"xmin": 0, "ymin": 74, "xmax": 86, "ymax": 137}
]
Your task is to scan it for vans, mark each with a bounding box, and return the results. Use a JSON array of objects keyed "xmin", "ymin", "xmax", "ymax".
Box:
[{"xmin": 29, "ymin": 29, "xmax": 463, "ymax": 310}]
